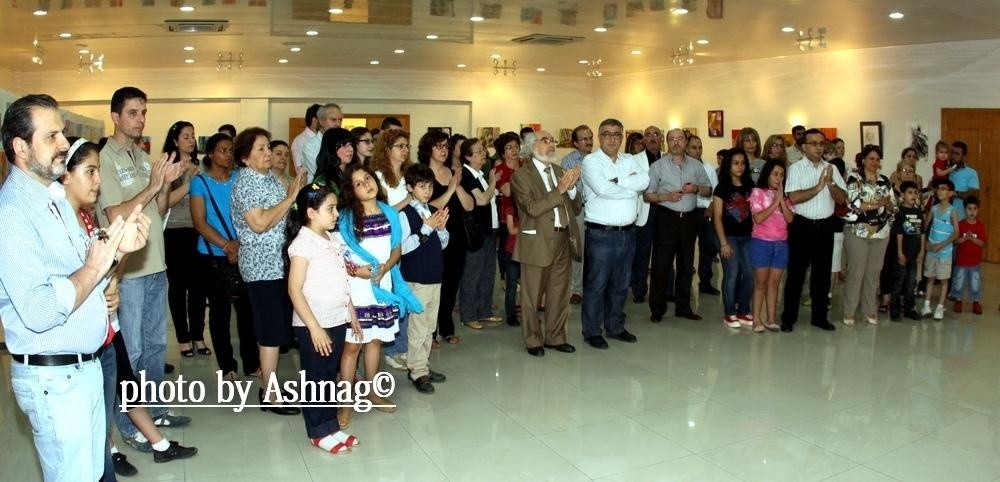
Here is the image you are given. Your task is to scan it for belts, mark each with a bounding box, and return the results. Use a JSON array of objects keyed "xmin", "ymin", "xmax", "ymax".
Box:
[
  {"xmin": 10, "ymin": 354, "xmax": 100, "ymax": 366},
  {"xmin": 677, "ymin": 212, "xmax": 687, "ymax": 218},
  {"xmin": 554, "ymin": 227, "xmax": 568, "ymax": 231},
  {"xmin": 587, "ymin": 222, "xmax": 633, "ymax": 231}
]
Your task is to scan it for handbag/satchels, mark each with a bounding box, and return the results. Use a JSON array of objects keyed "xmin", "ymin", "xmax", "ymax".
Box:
[{"xmin": 209, "ymin": 265, "xmax": 246, "ymax": 302}]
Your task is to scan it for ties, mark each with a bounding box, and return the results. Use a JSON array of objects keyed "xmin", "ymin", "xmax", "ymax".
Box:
[{"xmin": 544, "ymin": 167, "xmax": 568, "ymax": 226}]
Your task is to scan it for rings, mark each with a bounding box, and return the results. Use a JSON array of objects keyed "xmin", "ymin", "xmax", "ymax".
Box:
[{"xmin": 97, "ymin": 229, "xmax": 110, "ymax": 243}]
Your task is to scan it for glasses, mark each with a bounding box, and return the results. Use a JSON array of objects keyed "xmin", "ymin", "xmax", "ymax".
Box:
[
  {"xmin": 539, "ymin": 133, "xmax": 683, "ymax": 141},
  {"xmin": 359, "ymin": 138, "xmax": 450, "ymax": 150},
  {"xmin": 771, "ymin": 140, "xmax": 785, "ymax": 146},
  {"xmin": 216, "ymin": 147, "xmax": 233, "ymax": 155},
  {"xmin": 311, "ymin": 174, "xmax": 336, "ymax": 195}
]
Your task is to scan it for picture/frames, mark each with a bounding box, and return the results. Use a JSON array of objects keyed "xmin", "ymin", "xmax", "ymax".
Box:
[
  {"xmin": 858, "ymin": 120, "xmax": 883, "ymax": 161},
  {"xmin": 706, "ymin": 110, "xmax": 726, "ymax": 141}
]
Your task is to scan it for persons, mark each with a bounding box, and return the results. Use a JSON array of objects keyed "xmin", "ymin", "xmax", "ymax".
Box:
[
  {"xmin": 56, "ymin": 134, "xmax": 201, "ymax": 479},
  {"xmin": 93, "ymin": 80, "xmax": 194, "ymax": 454},
  {"xmin": 159, "ymin": 98, "xmax": 990, "ymax": 456},
  {"xmin": 136, "ymin": 134, "xmax": 176, "ymax": 374},
  {"xmin": 1, "ymin": 91, "xmax": 154, "ymax": 482}
]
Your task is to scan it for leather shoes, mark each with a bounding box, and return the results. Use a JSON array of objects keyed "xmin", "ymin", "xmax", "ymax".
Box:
[
  {"xmin": 632, "ymin": 283, "xmax": 720, "ymax": 323},
  {"xmin": 723, "ymin": 297, "xmax": 879, "ymax": 332},
  {"xmin": 180, "ymin": 341, "xmax": 301, "ymax": 415},
  {"xmin": 432, "ymin": 294, "xmax": 581, "ymax": 356},
  {"xmin": 611, "ymin": 330, "xmax": 636, "ymax": 342},
  {"xmin": 585, "ymin": 336, "xmax": 608, "ymax": 348}
]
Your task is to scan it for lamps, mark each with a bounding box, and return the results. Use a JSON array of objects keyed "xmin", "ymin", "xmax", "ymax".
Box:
[
  {"xmin": 69, "ymin": 46, "xmax": 108, "ymax": 78},
  {"xmin": 672, "ymin": 40, "xmax": 696, "ymax": 74},
  {"xmin": 798, "ymin": 26, "xmax": 828, "ymax": 55},
  {"xmin": 492, "ymin": 58, "xmax": 522, "ymax": 81},
  {"xmin": 586, "ymin": 60, "xmax": 603, "ymax": 82},
  {"xmin": 209, "ymin": 50, "xmax": 246, "ymax": 75}
]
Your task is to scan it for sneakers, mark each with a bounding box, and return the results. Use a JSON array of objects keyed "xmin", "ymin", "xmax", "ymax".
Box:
[
  {"xmin": 310, "ymin": 351, "xmax": 445, "ymax": 454},
  {"xmin": 878, "ymin": 284, "xmax": 982, "ymax": 321},
  {"xmin": 112, "ymin": 412, "xmax": 198, "ymax": 475}
]
